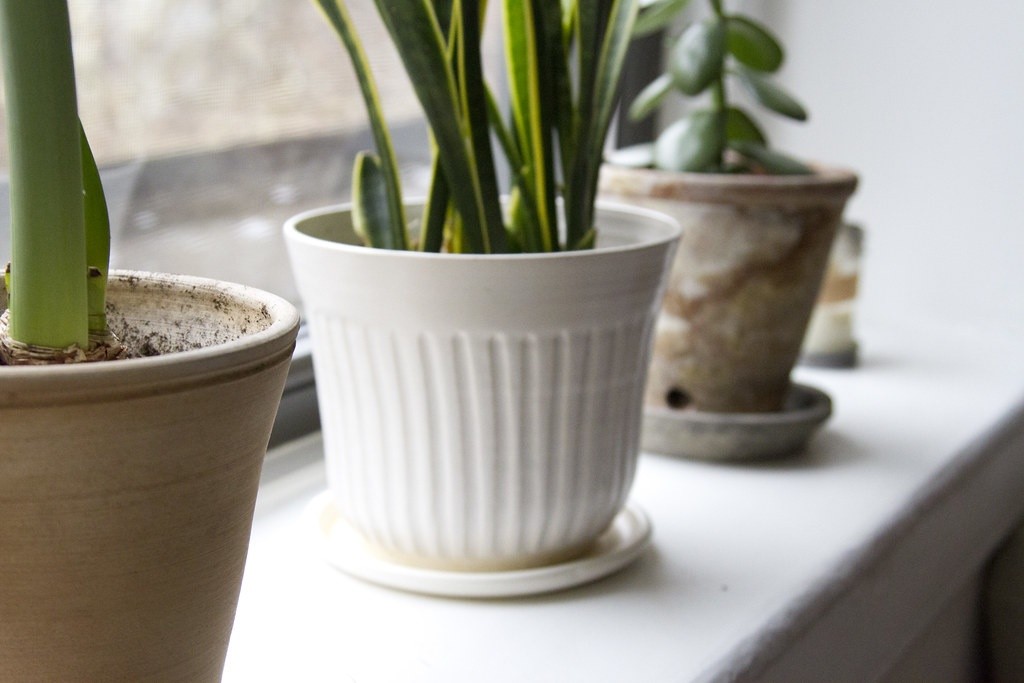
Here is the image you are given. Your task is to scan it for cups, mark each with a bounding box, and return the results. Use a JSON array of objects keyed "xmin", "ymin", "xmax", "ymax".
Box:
[{"xmin": 793, "ymin": 221, "xmax": 861, "ymax": 370}]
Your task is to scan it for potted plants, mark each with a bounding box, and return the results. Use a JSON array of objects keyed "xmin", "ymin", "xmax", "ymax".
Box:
[
  {"xmin": 284, "ymin": 0, "xmax": 684, "ymax": 572},
  {"xmin": 598, "ymin": 0, "xmax": 860, "ymax": 412},
  {"xmin": 0, "ymin": 0, "xmax": 300, "ymax": 683}
]
